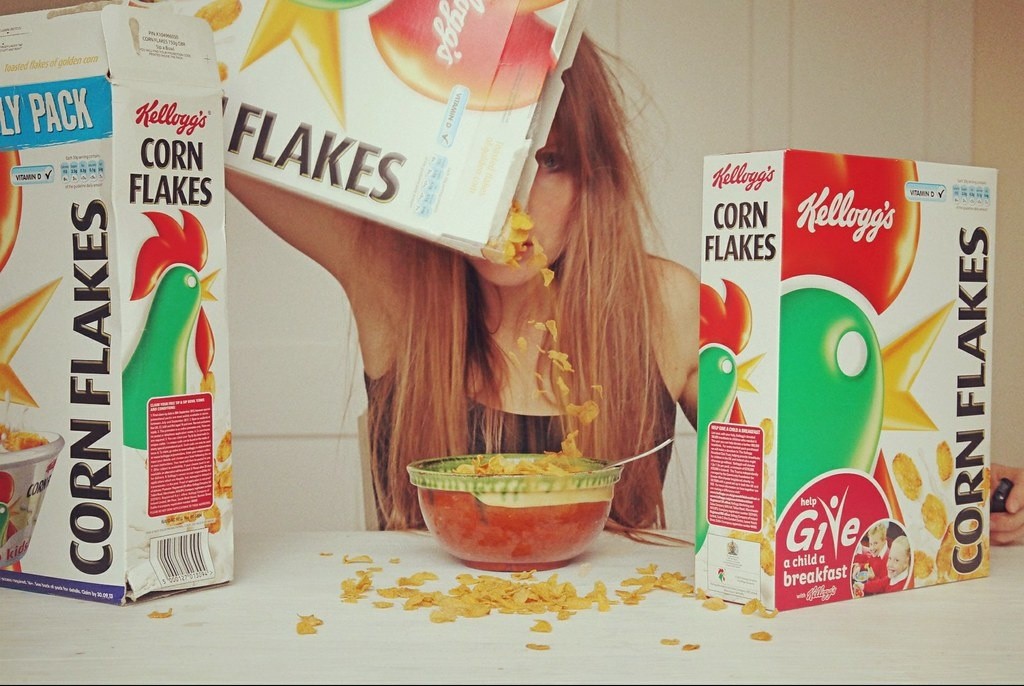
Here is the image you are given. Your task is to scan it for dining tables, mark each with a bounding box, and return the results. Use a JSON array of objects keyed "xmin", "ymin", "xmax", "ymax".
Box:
[{"xmin": 0, "ymin": 528, "xmax": 1023, "ymax": 686}]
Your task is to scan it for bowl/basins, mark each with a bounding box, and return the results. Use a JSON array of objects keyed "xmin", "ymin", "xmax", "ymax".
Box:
[
  {"xmin": 855, "ymin": 571, "xmax": 869, "ymax": 582},
  {"xmin": 404, "ymin": 450, "xmax": 625, "ymax": 574}
]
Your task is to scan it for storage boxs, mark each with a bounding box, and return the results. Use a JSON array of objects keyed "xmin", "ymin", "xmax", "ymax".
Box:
[
  {"xmin": 152, "ymin": 0, "xmax": 591, "ymax": 269},
  {"xmin": 1, "ymin": 1, "xmax": 239, "ymax": 607},
  {"xmin": 693, "ymin": 148, "xmax": 1001, "ymax": 612}
]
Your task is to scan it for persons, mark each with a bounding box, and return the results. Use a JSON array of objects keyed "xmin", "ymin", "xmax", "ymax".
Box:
[{"xmin": 222, "ymin": 28, "xmax": 1024, "ymax": 548}]
